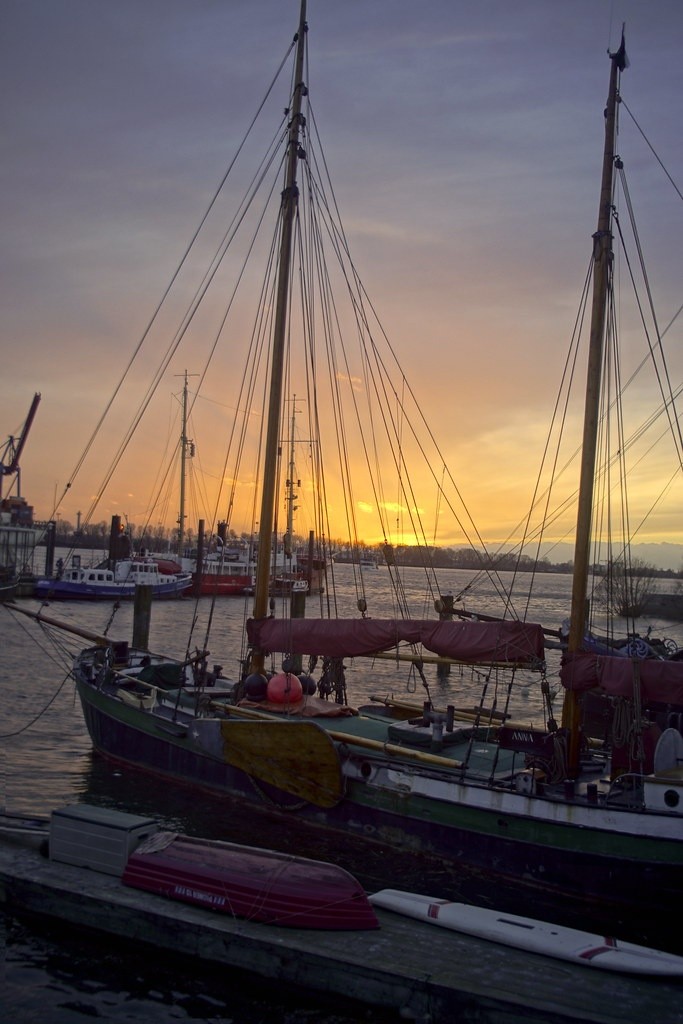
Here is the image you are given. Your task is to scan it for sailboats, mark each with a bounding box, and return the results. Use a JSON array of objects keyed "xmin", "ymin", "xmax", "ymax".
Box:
[{"xmin": 0, "ymin": 2, "xmax": 682, "ymax": 942}]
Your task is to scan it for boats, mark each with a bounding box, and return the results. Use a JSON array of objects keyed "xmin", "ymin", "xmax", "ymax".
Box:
[
  {"xmin": 182, "ymin": 533, "xmax": 327, "ymax": 598},
  {"xmin": 357, "ymin": 559, "xmax": 380, "ymax": 572},
  {"xmin": 34, "ymin": 557, "xmax": 195, "ymax": 599},
  {"xmin": 0, "ymin": 801, "xmax": 682, "ymax": 1024},
  {"xmin": 240, "ymin": 576, "xmax": 311, "ymax": 597}
]
